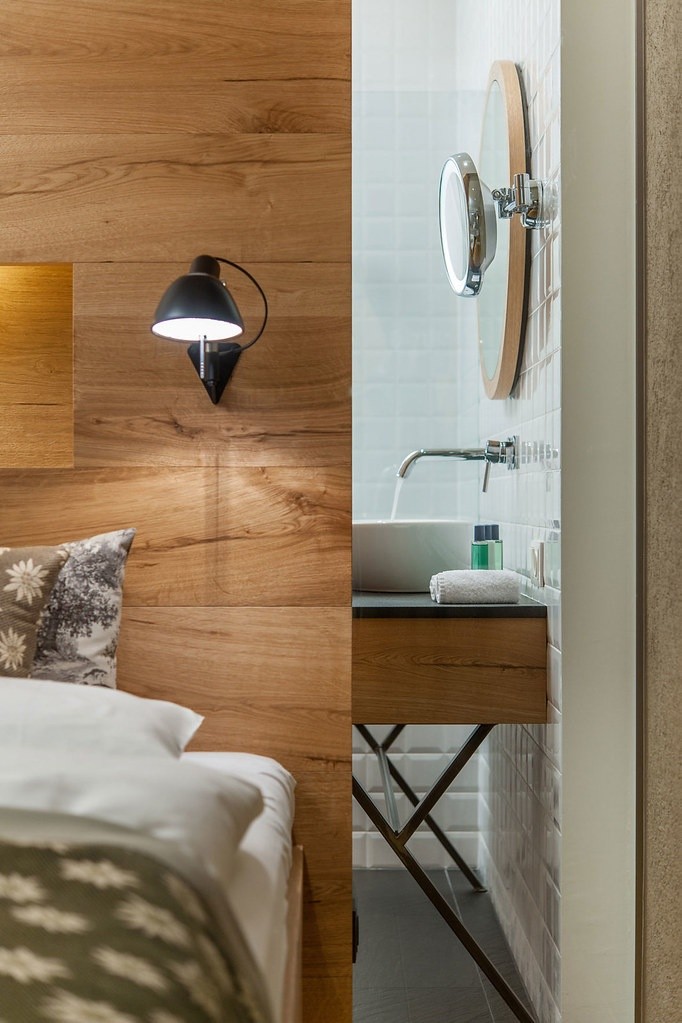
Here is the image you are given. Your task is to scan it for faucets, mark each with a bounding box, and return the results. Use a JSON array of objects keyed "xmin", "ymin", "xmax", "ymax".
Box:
[{"xmin": 397, "ymin": 435, "xmax": 519, "ymax": 493}]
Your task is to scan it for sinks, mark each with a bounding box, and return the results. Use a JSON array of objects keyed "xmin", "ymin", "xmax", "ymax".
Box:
[{"xmin": 352, "ymin": 520, "xmax": 477, "ymax": 592}]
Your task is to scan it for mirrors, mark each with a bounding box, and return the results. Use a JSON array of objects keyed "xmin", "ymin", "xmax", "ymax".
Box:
[{"xmin": 475, "ymin": 59, "xmax": 530, "ymax": 398}]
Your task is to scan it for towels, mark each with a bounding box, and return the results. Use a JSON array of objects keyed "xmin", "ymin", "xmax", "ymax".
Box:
[{"xmin": 429, "ymin": 571, "xmax": 522, "ymax": 603}]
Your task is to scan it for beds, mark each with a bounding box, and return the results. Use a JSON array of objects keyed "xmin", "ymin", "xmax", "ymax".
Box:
[{"xmin": 0, "ymin": 752, "xmax": 304, "ymax": 1023}]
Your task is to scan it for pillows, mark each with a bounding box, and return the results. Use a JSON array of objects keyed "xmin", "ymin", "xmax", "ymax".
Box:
[
  {"xmin": 32, "ymin": 527, "xmax": 137, "ymax": 690},
  {"xmin": 0, "ymin": 763, "xmax": 266, "ymax": 882},
  {"xmin": 0, "ymin": 676, "xmax": 217, "ymax": 878},
  {"xmin": 0, "ymin": 545, "xmax": 70, "ymax": 678}
]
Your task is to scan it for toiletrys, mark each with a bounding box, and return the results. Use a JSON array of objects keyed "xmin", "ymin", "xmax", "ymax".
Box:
[{"xmin": 472, "ymin": 524, "xmax": 504, "ymax": 568}]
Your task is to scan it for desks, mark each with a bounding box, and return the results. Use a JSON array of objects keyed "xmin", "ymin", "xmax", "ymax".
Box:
[{"xmin": 352, "ymin": 590, "xmax": 550, "ymax": 1023}]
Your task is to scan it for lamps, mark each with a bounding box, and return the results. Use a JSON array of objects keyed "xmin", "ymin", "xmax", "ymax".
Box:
[
  {"xmin": 439, "ymin": 152, "xmax": 545, "ymax": 304},
  {"xmin": 149, "ymin": 255, "xmax": 267, "ymax": 403}
]
